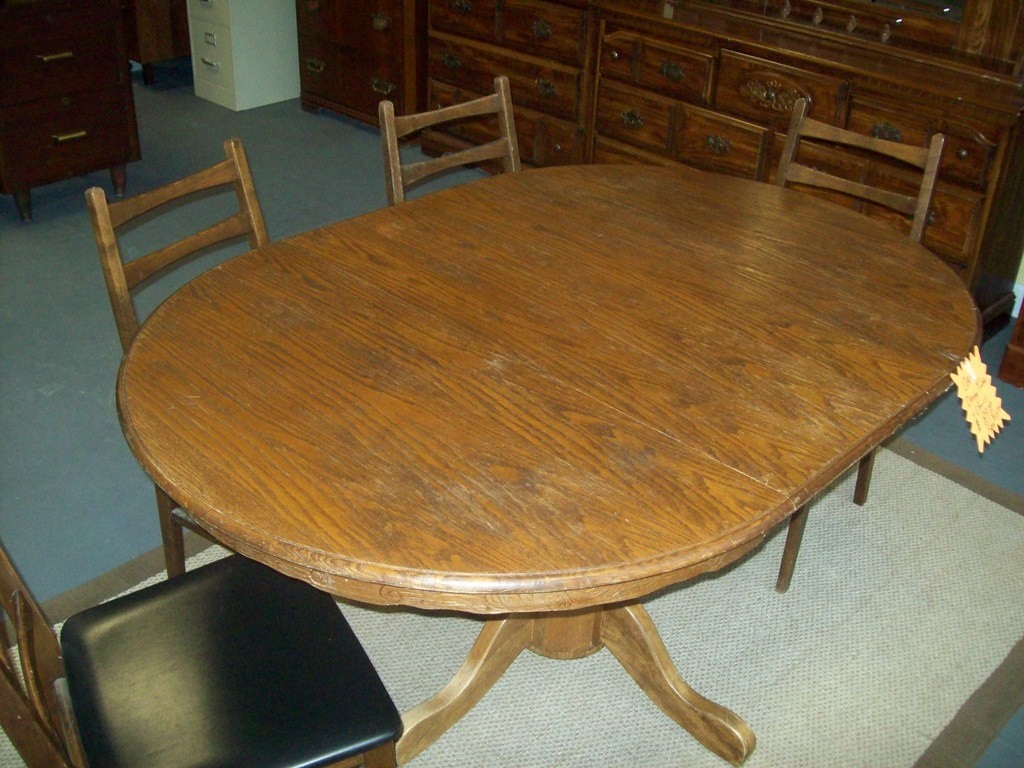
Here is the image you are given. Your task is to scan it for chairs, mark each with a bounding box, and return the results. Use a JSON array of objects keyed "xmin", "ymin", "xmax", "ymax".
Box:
[
  {"xmin": 775, "ymin": 98, "xmax": 944, "ymax": 592},
  {"xmin": 0, "ymin": 542, "xmax": 403, "ymax": 768},
  {"xmin": 379, "ymin": 75, "xmax": 518, "ymax": 205},
  {"xmin": 85, "ymin": 137, "xmax": 269, "ymax": 579}
]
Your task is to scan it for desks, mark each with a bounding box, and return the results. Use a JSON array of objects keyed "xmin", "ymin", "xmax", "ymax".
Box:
[{"xmin": 114, "ymin": 163, "xmax": 984, "ymax": 768}]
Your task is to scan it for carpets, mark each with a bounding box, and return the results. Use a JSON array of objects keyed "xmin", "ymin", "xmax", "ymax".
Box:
[{"xmin": 2, "ymin": 442, "xmax": 1024, "ymax": 768}]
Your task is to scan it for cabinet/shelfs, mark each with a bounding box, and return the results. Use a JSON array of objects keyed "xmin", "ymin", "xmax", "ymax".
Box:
[
  {"xmin": 0, "ymin": 0, "xmax": 142, "ymax": 223},
  {"xmin": 126, "ymin": 0, "xmax": 1024, "ymax": 391}
]
